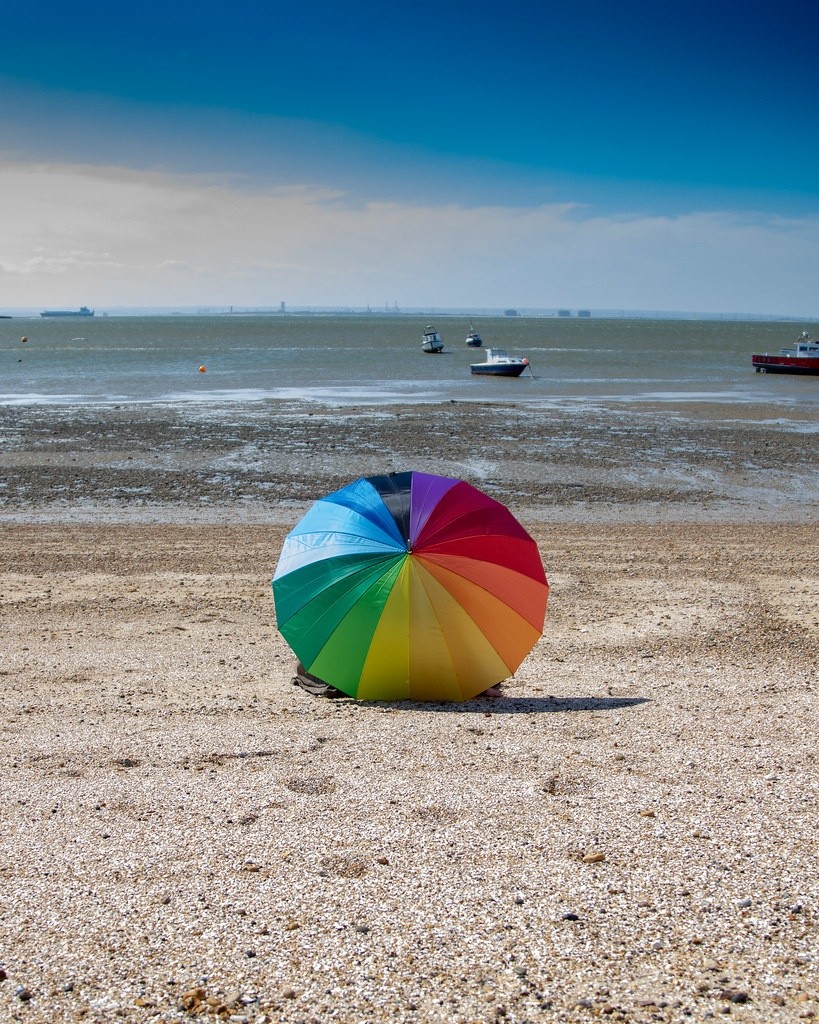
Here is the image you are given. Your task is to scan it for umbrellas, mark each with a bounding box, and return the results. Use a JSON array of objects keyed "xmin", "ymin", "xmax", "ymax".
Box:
[{"xmin": 271, "ymin": 471, "xmax": 550, "ymax": 704}]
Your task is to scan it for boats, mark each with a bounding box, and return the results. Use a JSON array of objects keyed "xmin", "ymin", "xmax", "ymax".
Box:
[
  {"xmin": 464, "ymin": 323, "xmax": 483, "ymax": 347},
  {"xmin": 420, "ymin": 323, "xmax": 445, "ymax": 353},
  {"xmin": 752, "ymin": 331, "xmax": 819, "ymax": 376},
  {"xmin": 39, "ymin": 306, "xmax": 95, "ymax": 317},
  {"xmin": 470, "ymin": 347, "xmax": 528, "ymax": 377}
]
